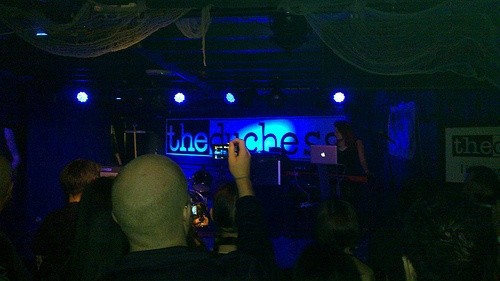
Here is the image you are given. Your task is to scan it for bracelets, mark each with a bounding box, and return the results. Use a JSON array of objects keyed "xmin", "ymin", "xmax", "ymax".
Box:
[{"xmin": 234, "ymin": 176, "xmax": 250, "ymax": 180}]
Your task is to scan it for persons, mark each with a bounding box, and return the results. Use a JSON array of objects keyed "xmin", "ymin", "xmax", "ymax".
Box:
[
  {"xmin": 293, "ymin": 166, "xmax": 500, "ymax": 281},
  {"xmin": 188, "ymin": 171, "xmax": 215, "ymax": 252},
  {"xmin": 0, "ymin": 155, "xmax": 130, "ymax": 281},
  {"xmin": 97, "ymin": 138, "xmax": 278, "ymax": 281},
  {"xmin": 335, "ymin": 122, "xmax": 372, "ymax": 175}
]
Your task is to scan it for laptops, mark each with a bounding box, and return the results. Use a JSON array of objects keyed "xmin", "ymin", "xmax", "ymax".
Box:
[{"xmin": 311, "ymin": 145, "xmax": 345, "ymax": 165}]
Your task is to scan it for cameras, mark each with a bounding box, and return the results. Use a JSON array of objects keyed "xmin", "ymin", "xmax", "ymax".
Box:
[
  {"xmin": 213, "ymin": 142, "xmax": 239, "ymax": 159},
  {"xmin": 191, "ymin": 203, "xmax": 205, "ymax": 223}
]
